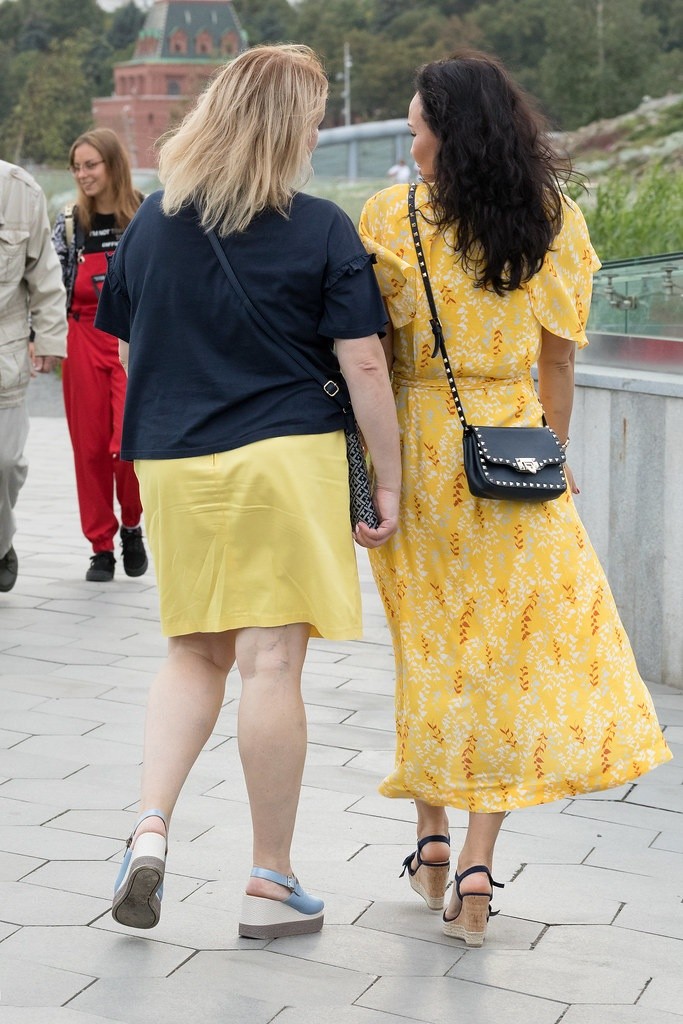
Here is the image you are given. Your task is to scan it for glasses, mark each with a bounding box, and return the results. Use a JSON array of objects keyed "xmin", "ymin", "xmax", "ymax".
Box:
[{"xmin": 67, "ymin": 159, "xmax": 106, "ymax": 177}]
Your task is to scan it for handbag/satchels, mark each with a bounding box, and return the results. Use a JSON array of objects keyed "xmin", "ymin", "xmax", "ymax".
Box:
[
  {"xmin": 461, "ymin": 420, "xmax": 570, "ymax": 502},
  {"xmin": 343, "ymin": 415, "xmax": 381, "ymax": 534}
]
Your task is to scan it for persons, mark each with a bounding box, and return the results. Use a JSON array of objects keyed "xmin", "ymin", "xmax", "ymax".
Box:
[
  {"xmin": 358, "ymin": 49, "xmax": 674, "ymax": 947},
  {"xmin": 29, "ymin": 128, "xmax": 152, "ymax": 582},
  {"xmin": 93, "ymin": 46, "xmax": 402, "ymax": 940},
  {"xmin": 0, "ymin": 158, "xmax": 68, "ymax": 593}
]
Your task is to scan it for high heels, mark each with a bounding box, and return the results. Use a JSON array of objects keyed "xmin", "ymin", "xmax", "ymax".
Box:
[
  {"xmin": 111, "ymin": 809, "xmax": 169, "ymax": 930},
  {"xmin": 238, "ymin": 867, "xmax": 326, "ymax": 937},
  {"xmin": 440, "ymin": 866, "xmax": 503, "ymax": 948},
  {"xmin": 404, "ymin": 834, "xmax": 452, "ymax": 912}
]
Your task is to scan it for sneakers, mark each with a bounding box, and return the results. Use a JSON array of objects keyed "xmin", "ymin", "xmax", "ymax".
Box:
[
  {"xmin": 121, "ymin": 525, "xmax": 149, "ymax": 576},
  {"xmin": 85, "ymin": 551, "xmax": 116, "ymax": 581}
]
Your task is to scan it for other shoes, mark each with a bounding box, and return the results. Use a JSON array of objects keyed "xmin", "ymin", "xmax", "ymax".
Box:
[{"xmin": 0, "ymin": 544, "xmax": 18, "ymax": 592}]
